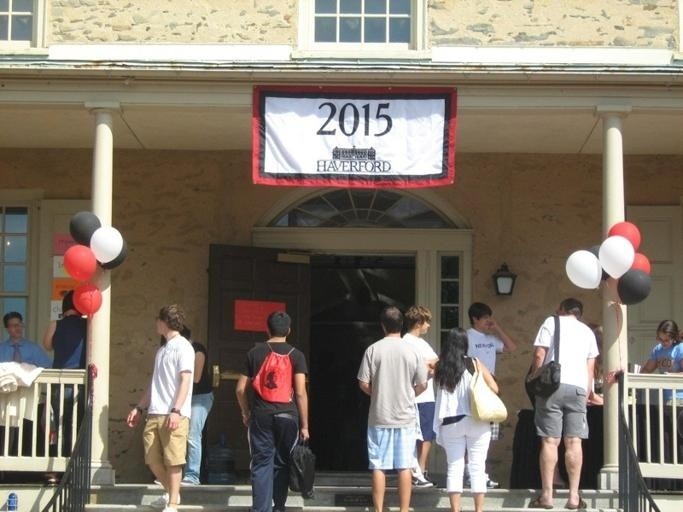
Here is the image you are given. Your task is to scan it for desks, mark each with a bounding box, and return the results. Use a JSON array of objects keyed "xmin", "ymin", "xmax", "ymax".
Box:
[{"xmin": 511, "ymin": 402, "xmax": 659, "ymax": 490}]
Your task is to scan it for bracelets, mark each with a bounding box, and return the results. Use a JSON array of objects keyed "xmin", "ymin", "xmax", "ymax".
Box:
[
  {"xmin": 171, "ymin": 408, "xmax": 181, "ymax": 415},
  {"xmin": 135, "ymin": 406, "xmax": 143, "ymax": 414},
  {"xmin": 241, "ymin": 412, "xmax": 250, "ymax": 417}
]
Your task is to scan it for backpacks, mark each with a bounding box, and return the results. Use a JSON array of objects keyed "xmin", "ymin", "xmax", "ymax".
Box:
[{"xmin": 251, "ymin": 343, "xmax": 295, "ymax": 403}]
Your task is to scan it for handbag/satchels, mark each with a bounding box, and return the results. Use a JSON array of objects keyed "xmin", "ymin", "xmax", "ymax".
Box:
[
  {"xmin": 469, "ymin": 372, "xmax": 507, "ymax": 422},
  {"xmin": 41, "ymin": 401, "xmax": 58, "ymax": 445},
  {"xmin": 288, "ymin": 437, "xmax": 316, "ymax": 493},
  {"xmin": 526, "ymin": 361, "xmax": 561, "ymax": 398}
]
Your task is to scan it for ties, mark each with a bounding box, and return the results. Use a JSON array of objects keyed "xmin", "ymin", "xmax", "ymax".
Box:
[{"xmin": 13, "ymin": 345, "xmax": 21, "ymax": 362}]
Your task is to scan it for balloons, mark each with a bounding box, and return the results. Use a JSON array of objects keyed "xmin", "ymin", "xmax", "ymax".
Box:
[
  {"xmin": 565, "ymin": 223, "xmax": 652, "ymax": 306},
  {"xmin": 64, "ymin": 211, "xmax": 127, "ymax": 319}
]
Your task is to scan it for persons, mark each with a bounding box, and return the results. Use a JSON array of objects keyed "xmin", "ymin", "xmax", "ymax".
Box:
[
  {"xmin": 236, "ymin": 311, "xmax": 310, "ymax": 511},
  {"xmin": 401, "ymin": 304, "xmax": 439, "ymax": 488},
  {"xmin": 0, "ymin": 311, "xmax": 53, "ymax": 483},
  {"xmin": 640, "ymin": 321, "xmax": 683, "ymax": 408},
  {"xmin": 587, "ymin": 327, "xmax": 604, "ymax": 406},
  {"xmin": 42, "ymin": 288, "xmax": 88, "ymax": 486},
  {"xmin": 152, "ymin": 323, "xmax": 214, "ymax": 486},
  {"xmin": 126, "ymin": 303, "xmax": 195, "ymax": 511},
  {"xmin": 464, "ymin": 302, "xmax": 517, "ymax": 490},
  {"xmin": 356, "ymin": 305, "xmax": 429, "ymax": 511},
  {"xmin": 528, "ymin": 297, "xmax": 600, "ymax": 509},
  {"xmin": 433, "ymin": 328, "xmax": 499, "ymax": 512}
]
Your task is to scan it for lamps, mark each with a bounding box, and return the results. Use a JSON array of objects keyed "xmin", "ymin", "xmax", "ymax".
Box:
[{"xmin": 491, "ymin": 261, "xmax": 517, "ymax": 296}]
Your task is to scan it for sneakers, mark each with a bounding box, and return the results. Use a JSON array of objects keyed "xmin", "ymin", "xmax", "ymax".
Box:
[
  {"xmin": 151, "ymin": 492, "xmax": 180, "ymax": 512},
  {"xmin": 45, "ymin": 474, "xmax": 59, "ymax": 483},
  {"xmin": 181, "ymin": 480, "xmax": 199, "ymax": 485},
  {"xmin": 412, "ymin": 471, "xmax": 437, "ymax": 487},
  {"xmin": 466, "ymin": 475, "xmax": 498, "ymax": 487}
]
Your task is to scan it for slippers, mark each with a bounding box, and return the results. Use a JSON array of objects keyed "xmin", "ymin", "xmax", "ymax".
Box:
[
  {"xmin": 567, "ymin": 497, "xmax": 586, "ymax": 509},
  {"xmin": 528, "ymin": 496, "xmax": 552, "ymax": 508}
]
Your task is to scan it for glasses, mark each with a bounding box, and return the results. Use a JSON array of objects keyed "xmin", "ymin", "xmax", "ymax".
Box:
[{"xmin": 656, "ymin": 337, "xmax": 674, "ymax": 344}]
